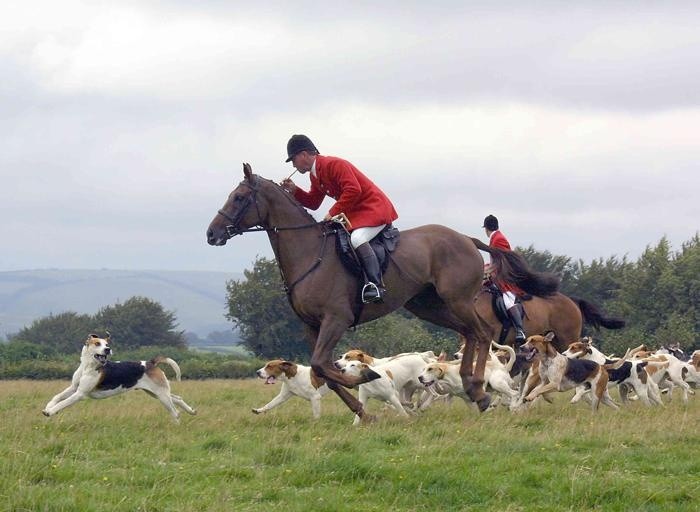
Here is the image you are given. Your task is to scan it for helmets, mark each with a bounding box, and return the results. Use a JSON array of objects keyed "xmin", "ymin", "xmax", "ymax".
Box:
[
  {"xmin": 286, "ymin": 135, "xmax": 320, "ymax": 162},
  {"xmin": 482, "ymin": 214, "xmax": 498, "ymax": 229}
]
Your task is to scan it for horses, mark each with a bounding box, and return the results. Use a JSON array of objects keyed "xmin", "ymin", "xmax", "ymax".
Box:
[
  {"xmin": 204, "ymin": 160, "xmax": 562, "ymax": 427},
  {"xmin": 474, "ymin": 281, "xmax": 626, "ymax": 354}
]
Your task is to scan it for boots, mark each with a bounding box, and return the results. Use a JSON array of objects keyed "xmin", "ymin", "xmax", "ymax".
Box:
[
  {"xmin": 507, "ymin": 305, "xmax": 524, "ymax": 341},
  {"xmin": 354, "ymin": 241, "xmax": 385, "ymax": 304}
]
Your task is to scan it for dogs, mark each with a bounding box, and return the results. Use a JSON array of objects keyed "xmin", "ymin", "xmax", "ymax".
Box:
[
  {"xmin": 335, "ymin": 331, "xmax": 700, "ymax": 427},
  {"xmin": 251, "ymin": 357, "xmax": 333, "ymax": 420},
  {"xmin": 42, "ymin": 330, "xmax": 197, "ymax": 428}
]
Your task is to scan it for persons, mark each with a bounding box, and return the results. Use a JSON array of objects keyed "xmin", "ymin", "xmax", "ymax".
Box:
[
  {"xmin": 478, "ymin": 215, "xmax": 526, "ymax": 343},
  {"xmin": 280, "ymin": 134, "xmax": 398, "ymax": 303}
]
